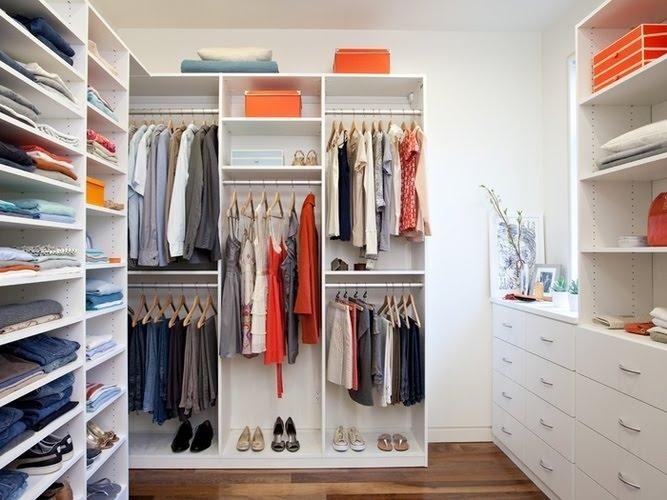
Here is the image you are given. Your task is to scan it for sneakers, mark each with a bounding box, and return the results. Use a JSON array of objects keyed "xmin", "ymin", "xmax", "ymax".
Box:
[
  {"xmin": 333, "ymin": 426, "xmax": 348, "ymax": 451},
  {"xmin": 87, "ymin": 449, "xmax": 102, "ymax": 465},
  {"xmin": 7, "ymin": 434, "xmax": 74, "ymax": 476},
  {"xmin": 347, "ymin": 427, "xmax": 365, "ymax": 451},
  {"xmin": 37, "ymin": 480, "xmax": 73, "ymax": 500}
]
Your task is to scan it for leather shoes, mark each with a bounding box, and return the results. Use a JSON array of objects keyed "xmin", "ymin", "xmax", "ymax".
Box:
[
  {"xmin": 170, "ymin": 420, "xmax": 193, "ymax": 452},
  {"xmin": 190, "ymin": 420, "xmax": 213, "ymax": 451},
  {"xmin": 237, "ymin": 426, "xmax": 250, "ymax": 451},
  {"xmin": 252, "ymin": 426, "xmax": 264, "ymax": 450}
]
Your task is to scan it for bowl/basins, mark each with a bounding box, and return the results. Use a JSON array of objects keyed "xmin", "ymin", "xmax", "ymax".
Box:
[{"xmin": 616, "ymin": 235, "xmax": 645, "ymax": 247}]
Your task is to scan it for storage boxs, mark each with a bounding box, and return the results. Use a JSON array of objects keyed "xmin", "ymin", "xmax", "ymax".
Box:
[
  {"xmin": 333, "ymin": 47, "xmax": 392, "ymax": 74},
  {"xmin": 244, "ymin": 90, "xmax": 304, "ymax": 117}
]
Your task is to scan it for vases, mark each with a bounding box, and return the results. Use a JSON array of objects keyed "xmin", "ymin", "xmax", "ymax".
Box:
[{"xmin": 520, "ymin": 261, "xmax": 532, "ymax": 294}]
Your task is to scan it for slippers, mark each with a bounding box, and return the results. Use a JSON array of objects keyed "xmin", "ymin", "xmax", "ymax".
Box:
[
  {"xmin": 393, "ymin": 434, "xmax": 408, "ymax": 451},
  {"xmin": 377, "ymin": 433, "xmax": 393, "ymax": 451},
  {"xmin": 87, "ymin": 477, "xmax": 121, "ymax": 500}
]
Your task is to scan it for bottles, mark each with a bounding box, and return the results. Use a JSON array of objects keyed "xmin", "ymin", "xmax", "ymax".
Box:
[{"xmin": 534, "ymin": 278, "xmax": 543, "ymax": 304}]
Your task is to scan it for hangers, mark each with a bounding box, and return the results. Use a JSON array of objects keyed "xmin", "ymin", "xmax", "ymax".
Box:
[
  {"xmin": 127, "ymin": 281, "xmax": 217, "ymax": 330},
  {"xmin": 327, "ymin": 106, "xmax": 428, "ymax": 155},
  {"xmin": 224, "ymin": 176, "xmax": 316, "ymax": 221},
  {"xmin": 129, "ymin": 103, "xmax": 218, "ymax": 130},
  {"xmin": 328, "ymin": 282, "xmax": 423, "ymax": 330}
]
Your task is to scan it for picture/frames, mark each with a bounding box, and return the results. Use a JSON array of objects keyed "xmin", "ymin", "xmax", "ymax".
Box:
[
  {"xmin": 530, "ymin": 263, "xmax": 557, "ymax": 297},
  {"xmin": 488, "ymin": 211, "xmax": 547, "ymax": 292}
]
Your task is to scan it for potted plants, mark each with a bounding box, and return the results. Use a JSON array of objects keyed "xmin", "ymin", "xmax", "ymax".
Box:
[
  {"xmin": 567, "ymin": 280, "xmax": 577, "ymax": 312},
  {"xmin": 550, "ymin": 277, "xmax": 568, "ymax": 308}
]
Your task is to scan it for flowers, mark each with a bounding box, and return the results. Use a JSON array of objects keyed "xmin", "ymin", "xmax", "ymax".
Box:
[{"xmin": 481, "ymin": 183, "xmax": 523, "ymax": 263}]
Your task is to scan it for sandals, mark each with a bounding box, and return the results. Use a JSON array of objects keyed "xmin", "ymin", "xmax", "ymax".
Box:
[
  {"xmin": 86, "ymin": 421, "xmax": 119, "ymax": 448},
  {"xmin": 271, "ymin": 417, "xmax": 285, "ymax": 451},
  {"xmin": 286, "ymin": 417, "xmax": 299, "ymax": 451},
  {"xmin": 292, "ymin": 151, "xmax": 318, "ymax": 166}
]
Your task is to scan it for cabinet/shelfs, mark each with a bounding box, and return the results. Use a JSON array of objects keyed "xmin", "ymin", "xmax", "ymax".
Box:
[
  {"xmin": 130, "ymin": 55, "xmax": 429, "ymax": 470},
  {"xmin": 0, "ymin": 1, "xmax": 129, "ymax": 500}
]
